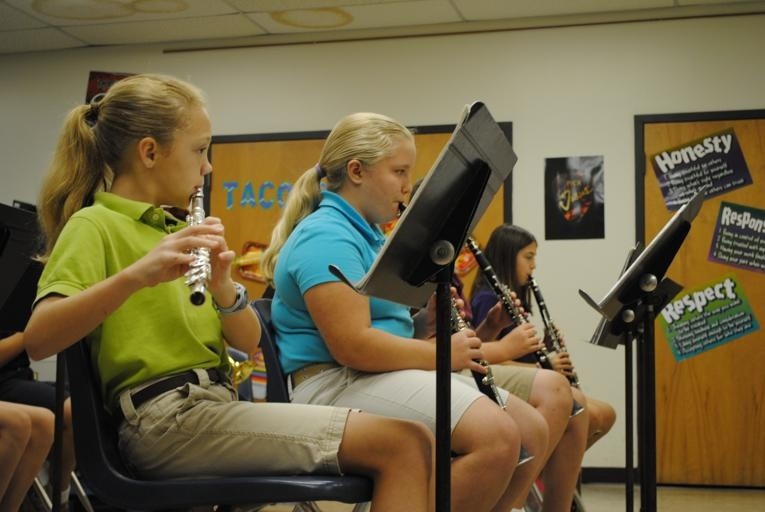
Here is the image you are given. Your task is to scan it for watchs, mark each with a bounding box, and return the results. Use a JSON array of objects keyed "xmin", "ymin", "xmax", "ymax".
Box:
[{"xmin": 212, "ymin": 278, "xmax": 249, "ymax": 315}]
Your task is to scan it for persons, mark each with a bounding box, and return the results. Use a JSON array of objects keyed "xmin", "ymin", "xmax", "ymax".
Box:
[
  {"xmin": 19, "ymin": 73, "xmax": 435, "ymax": 511},
  {"xmin": 0, "ymin": 218, "xmax": 79, "ymax": 512},
  {"xmin": 468, "ymin": 227, "xmax": 617, "ymax": 510},
  {"xmin": 255, "ymin": 112, "xmax": 553, "ymax": 511},
  {"xmin": 0, "ymin": 330, "xmax": 58, "ymax": 512},
  {"xmin": 404, "ymin": 176, "xmax": 590, "ymax": 509}
]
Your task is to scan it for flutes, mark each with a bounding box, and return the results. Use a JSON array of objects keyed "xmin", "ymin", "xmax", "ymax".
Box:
[{"xmin": 185, "ymin": 188, "xmax": 212, "ymax": 306}]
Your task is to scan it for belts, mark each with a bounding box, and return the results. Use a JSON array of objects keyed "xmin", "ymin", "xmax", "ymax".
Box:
[
  {"xmin": 109, "ymin": 366, "xmax": 233, "ymax": 430},
  {"xmin": 290, "ymin": 360, "xmax": 339, "ymax": 389}
]
